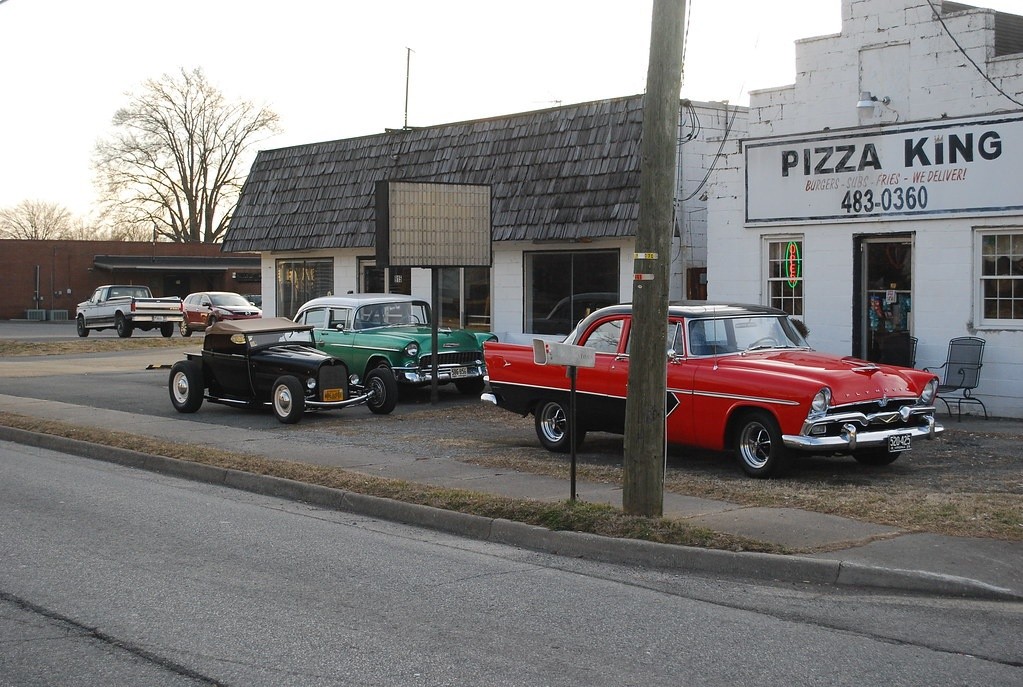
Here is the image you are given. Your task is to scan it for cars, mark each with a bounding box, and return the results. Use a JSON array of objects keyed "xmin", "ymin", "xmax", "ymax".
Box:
[
  {"xmin": 532, "ymin": 292, "xmax": 622, "ymax": 335},
  {"xmin": 168, "ymin": 316, "xmax": 399, "ymax": 420},
  {"xmin": 292, "ymin": 293, "xmax": 500, "ymax": 415},
  {"xmin": 179, "ymin": 291, "xmax": 264, "ymax": 338}
]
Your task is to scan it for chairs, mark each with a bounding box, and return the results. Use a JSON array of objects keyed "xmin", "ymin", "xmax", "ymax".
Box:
[
  {"xmin": 220, "ymin": 316, "xmax": 729, "ymax": 356},
  {"xmin": 867, "ymin": 331, "xmax": 918, "ymax": 368},
  {"xmin": 922, "ymin": 336, "xmax": 988, "ymax": 423}
]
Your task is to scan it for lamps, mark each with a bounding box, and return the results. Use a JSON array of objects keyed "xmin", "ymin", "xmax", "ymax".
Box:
[{"xmin": 856, "ymin": 91, "xmax": 891, "ymax": 109}]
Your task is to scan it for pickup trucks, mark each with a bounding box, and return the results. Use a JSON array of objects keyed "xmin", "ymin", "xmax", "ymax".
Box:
[
  {"xmin": 74, "ymin": 285, "xmax": 185, "ymax": 338},
  {"xmin": 480, "ymin": 299, "xmax": 945, "ymax": 479}
]
